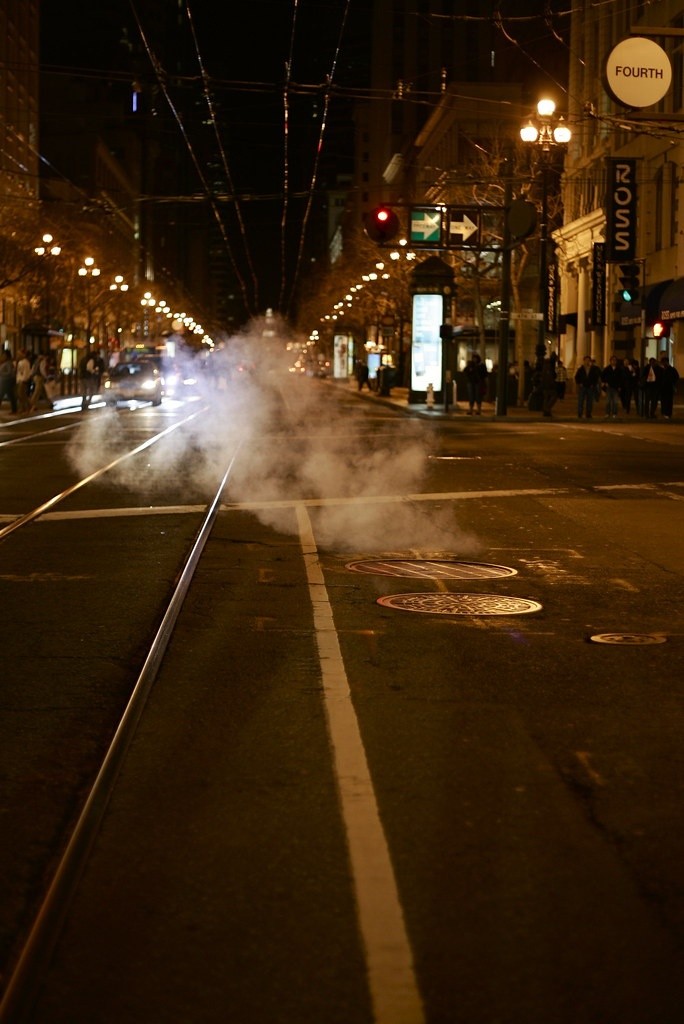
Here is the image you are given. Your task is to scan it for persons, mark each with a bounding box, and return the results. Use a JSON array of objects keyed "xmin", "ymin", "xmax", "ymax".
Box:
[
  {"xmin": 79, "ymin": 351, "xmax": 99, "ymax": 405},
  {"xmin": 539, "ymin": 357, "xmax": 558, "ymax": 415},
  {"xmin": 600, "ymin": 351, "xmax": 680, "ymax": 419},
  {"xmin": 358, "ymin": 363, "xmax": 372, "ymax": 392},
  {"xmin": 0, "ymin": 349, "xmax": 56, "ymax": 414},
  {"xmin": 96, "ymin": 352, "xmax": 105, "ymax": 387},
  {"xmin": 555, "ymin": 360, "xmax": 568, "ymax": 400},
  {"xmin": 464, "ymin": 354, "xmax": 487, "ymax": 415},
  {"xmin": 524, "ymin": 359, "xmax": 533, "ymax": 401},
  {"xmin": 575, "ymin": 356, "xmax": 600, "ymax": 418}
]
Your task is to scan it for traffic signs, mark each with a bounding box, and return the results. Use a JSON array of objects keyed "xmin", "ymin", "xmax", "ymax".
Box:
[
  {"xmin": 407, "ymin": 207, "xmax": 441, "ymax": 243},
  {"xmin": 449, "ymin": 209, "xmax": 478, "ymax": 244}
]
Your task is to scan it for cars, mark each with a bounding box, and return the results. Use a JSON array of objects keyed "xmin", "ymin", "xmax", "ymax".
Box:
[
  {"xmin": 104, "ymin": 361, "xmax": 163, "ymax": 407},
  {"xmin": 164, "ymin": 362, "xmax": 246, "ymax": 398}
]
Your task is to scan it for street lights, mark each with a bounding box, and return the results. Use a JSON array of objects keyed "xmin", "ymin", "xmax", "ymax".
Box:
[
  {"xmin": 303, "ymin": 237, "xmax": 417, "ymax": 386},
  {"xmin": 521, "ymin": 97, "xmax": 571, "ymax": 416},
  {"xmin": 110, "ymin": 275, "xmax": 215, "ymax": 353},
  {"xmin": 34, "ymin": 234, "xmax": 61, "ymax": 349},
  {"xmin": 78, "ymin": 256, "xmax": 100, "ymax": 356}
]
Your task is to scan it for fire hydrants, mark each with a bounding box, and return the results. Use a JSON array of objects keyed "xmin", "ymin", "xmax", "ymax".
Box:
[{"xmin": 425, "ymin": 383, "xmax": 435, "ymax": 408}]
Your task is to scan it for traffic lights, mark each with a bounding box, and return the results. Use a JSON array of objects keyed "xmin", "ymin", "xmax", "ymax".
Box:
[
  {"xmin": 619, "ymin": 265, "xmax": 640, "ymax": 304},
  {"xmin": 367, "ymin": 208, "xmax": 399, "ymax": 242}
]
